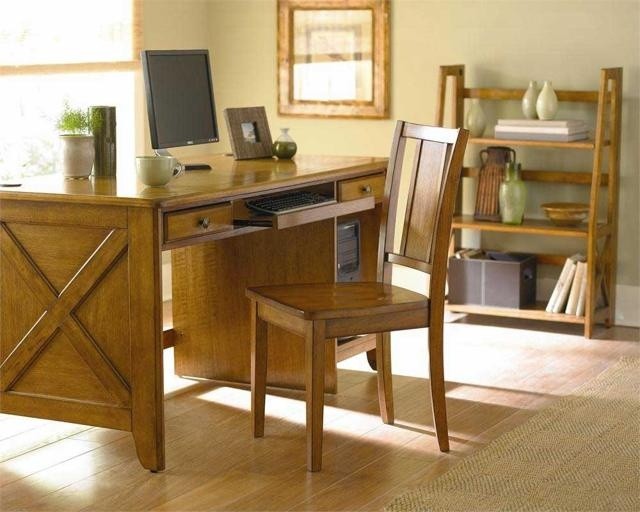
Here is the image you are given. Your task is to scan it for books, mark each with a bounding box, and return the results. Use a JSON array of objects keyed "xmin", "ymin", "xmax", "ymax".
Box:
[
  {"xmin": 494, "ymin": 119, "xmax": 590, "ymax": 142},
  {"xmin": 547, "ymin": 253, "xmax": 607, "ymax": 316},
  {"xmin": 456, "ymin": 248, "xmax": 530, "ymax": 280}
]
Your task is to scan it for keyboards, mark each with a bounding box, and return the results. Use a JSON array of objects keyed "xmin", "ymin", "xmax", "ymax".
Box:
[{"xmin": 245, "ymin": 189, "xmax": 337, "ymax": 215}]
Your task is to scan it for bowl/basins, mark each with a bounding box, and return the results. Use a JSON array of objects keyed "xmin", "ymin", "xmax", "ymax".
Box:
[{"xmin": 540, "ymin": 203, "xmax": 591, "ymax": 224}]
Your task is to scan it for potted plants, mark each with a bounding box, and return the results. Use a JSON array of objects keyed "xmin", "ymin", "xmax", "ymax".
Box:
[{"xmin": 40, "ymin": 92, "xmax": 116, "ymax": 179}]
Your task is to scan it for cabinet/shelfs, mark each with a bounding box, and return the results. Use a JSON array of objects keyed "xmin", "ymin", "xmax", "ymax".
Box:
[{"xmin": 433, "ymin": 65, "xmax": 623, "ymax": 338}]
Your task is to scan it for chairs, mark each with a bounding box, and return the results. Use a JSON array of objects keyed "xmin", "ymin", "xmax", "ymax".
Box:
[{"xmin": 243, "ymin": 120, "xmax": 470, "ymax": 472}]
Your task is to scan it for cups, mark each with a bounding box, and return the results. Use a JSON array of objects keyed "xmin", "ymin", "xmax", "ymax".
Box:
[
  {"xmin": 88, "ymin": 105, "xmax": 118, "ymax": 180},
  {"xmin": 134, "ymin": 155, "xmax": 182, "ymax": 187}
]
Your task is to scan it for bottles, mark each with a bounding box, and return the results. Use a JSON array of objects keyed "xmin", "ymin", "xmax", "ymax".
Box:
[
  {"xmin": 519, "ymin": 77, "xmax": 558, "ymax": 120},
  {"xmin": 475, "ymin": 147, "xmax": 527, "ymax": 225},
  {"xmin": 465, "ymin": 98, "xmax": 484, "ymax": 136},
  {"xmin": 272, "ymin": 127, "xmax": 297, "ymax": 160}
]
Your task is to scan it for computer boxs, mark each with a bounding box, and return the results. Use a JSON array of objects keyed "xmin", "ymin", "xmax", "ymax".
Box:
[{"xmin": 336, "ymin": 218, "xmax": 362, "ymax": 346}]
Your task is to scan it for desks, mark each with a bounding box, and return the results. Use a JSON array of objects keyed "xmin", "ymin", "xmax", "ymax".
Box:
[{"xmin": 0, "ymin": 155, "xmax": 388, "ymax": 471}]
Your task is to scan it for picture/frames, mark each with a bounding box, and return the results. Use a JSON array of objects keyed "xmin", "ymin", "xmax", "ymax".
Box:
[
  {"xmin": 276, "ymin": 0, "xmax": 389, "ymax": 118},
  {"xmin": 223, "ymin": 106, "xmax": 273, "ymax": 161}
]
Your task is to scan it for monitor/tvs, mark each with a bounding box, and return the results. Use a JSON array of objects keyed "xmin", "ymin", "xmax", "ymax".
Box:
[{"xmin": 141, "ymin": 48, "xmax": 220, "ymax": 175}]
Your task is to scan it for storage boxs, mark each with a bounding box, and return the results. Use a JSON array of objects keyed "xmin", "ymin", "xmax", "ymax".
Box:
[{"xmin": 449, "ymin": 249, "xmax": 535, "ymax": 309}]
